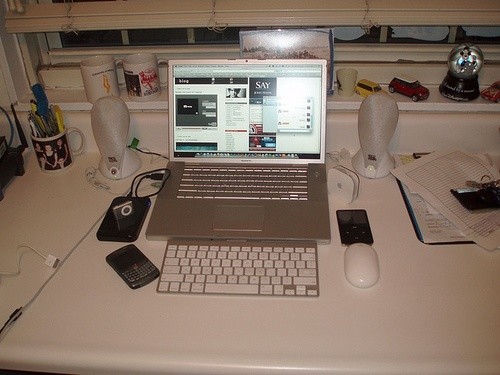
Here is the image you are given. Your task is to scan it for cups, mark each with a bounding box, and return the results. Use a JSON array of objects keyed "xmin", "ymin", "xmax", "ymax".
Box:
[
  {"xmin": 122, "ymin": 54, "xmax": 168, "ymax": 101},
  {"xmin": 336, "ymin": 69, "xmax": 359, "ymax": 96},
  {"xmin": 30, "ymin": 127, "xmax": 86, "ymax": 174},
  {"xmin": 80, "ymin": 55, "xmax": 121, "ymax": 103}
]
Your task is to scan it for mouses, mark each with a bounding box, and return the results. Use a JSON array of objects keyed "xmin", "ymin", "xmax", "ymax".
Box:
[{"xmin": 345, "ymin": 242, "xmax": 380, "ymax": 287}]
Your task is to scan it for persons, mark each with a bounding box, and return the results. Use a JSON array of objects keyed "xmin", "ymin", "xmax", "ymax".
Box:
[
  {"xmin": 34, "ymin": 143, "xmax": 45, "ymax": 169},
  {"xmin": 56, "ymin": 140, "xmax": 70, "ymax": 160},
  {"xmin": 44, "ymin": 144, "xmax": 58, "ymax": 170},
  {"xmin": 57, "ymin": 156, "xmax": 66, "ymax": 170},
  {"xmin": 227, "ymin": 88, "xmax": 241, "ymax": 98}
]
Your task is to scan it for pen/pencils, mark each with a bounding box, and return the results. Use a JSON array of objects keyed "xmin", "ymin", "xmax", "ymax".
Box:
[{"xmin": 28, "ymin": 100, "xmax": 64, "ymax": 137}]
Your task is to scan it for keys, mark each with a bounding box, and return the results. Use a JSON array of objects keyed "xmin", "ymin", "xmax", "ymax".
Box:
[{"xmin": 450, "ymin": 175, "xmax": 500, "ymax": 210}]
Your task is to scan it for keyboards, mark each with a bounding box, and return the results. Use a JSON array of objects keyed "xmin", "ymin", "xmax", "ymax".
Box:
[{"xmin": 156, "ymin": 239, "xmax": 321, "ymax": 298}]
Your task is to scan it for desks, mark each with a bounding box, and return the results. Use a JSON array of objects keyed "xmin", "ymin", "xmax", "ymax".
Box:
[{"xmin": 0, "ymin": 151, "xmax": 500, "ymax": 372}]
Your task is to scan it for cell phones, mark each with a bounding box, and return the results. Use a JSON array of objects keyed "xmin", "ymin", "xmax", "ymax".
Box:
[{"xmin": 105, "ymin": 244, "xmax": 160, "ymax": 289}]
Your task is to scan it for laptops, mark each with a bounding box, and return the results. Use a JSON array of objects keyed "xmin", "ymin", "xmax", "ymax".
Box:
[{"xmin": 146, "ymin": 57, "xmax": 332, "ymax": 245}]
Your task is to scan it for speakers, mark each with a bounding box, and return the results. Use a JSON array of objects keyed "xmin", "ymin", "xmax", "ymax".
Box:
[
  {"xmin": 90, "ymin": 96, "xmax": 142, "ymax": 180},
  {"xmin": 351, "ymin": 94, "xmax": 399, "ymax": 180}
]
